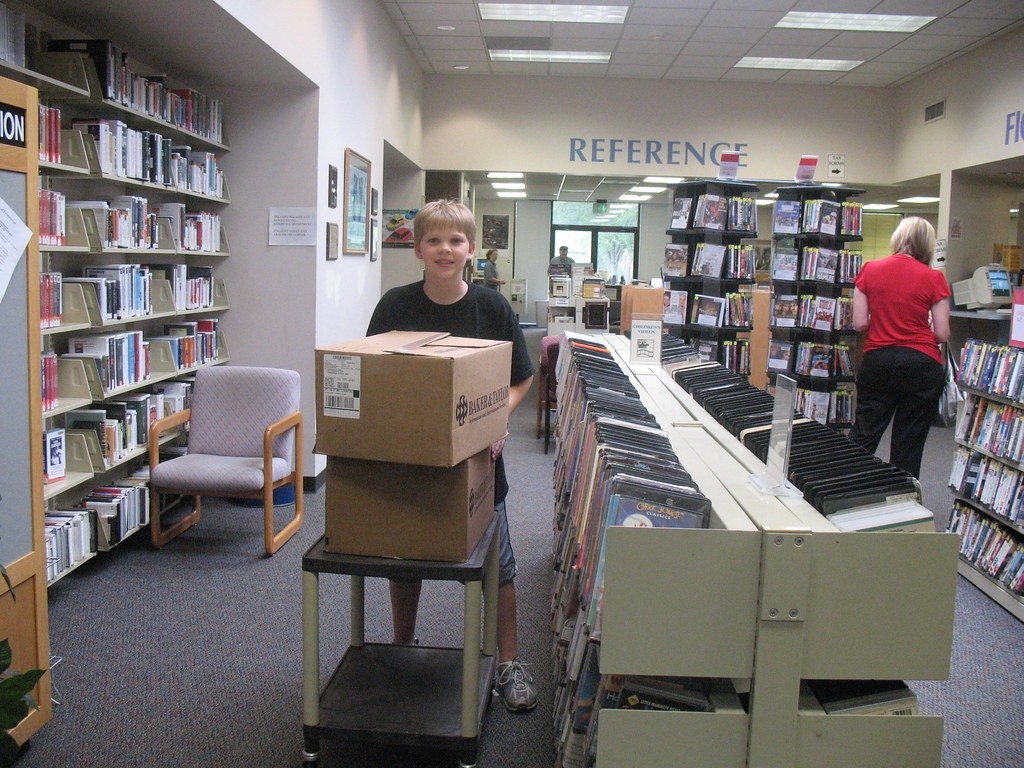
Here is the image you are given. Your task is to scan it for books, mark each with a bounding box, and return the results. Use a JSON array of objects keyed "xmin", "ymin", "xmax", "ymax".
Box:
[
  {"xmin": 0, "ymin": 1, "xmax": 26, "ymax": 67},
  {"xmin": 945, "ymin": 333, "xmax": 1024, "ymax": 615},
  {"xmin": 25, "ymin": 21, "xmax": 224, "ymax": 586},
  {"xmin": 555, "ymin": 171, "xmax": 933, "ymax": 723}
]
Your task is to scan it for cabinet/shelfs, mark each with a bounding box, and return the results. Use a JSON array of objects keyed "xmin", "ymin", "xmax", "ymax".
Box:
[
  {"xmin": 660, "ymin": 178, "xmax": 760, "ymax": 383},
  {"xmin": 301, "ymin": 506, "xmax": 500, "ymax": 768},
  {"xmin": 547, "ymin": 330, "xmax": 960, "ymax": 768},
  {"xmin": 0, "ymin": 51, "xmax": 228, "ymax": 591},
  {"xmin": 765, "ymin": 185, "xmax": 864, "ymax": 436},
  {"xmin": 945, "ymin": 346, "xmax": 1024, "ymax": 624}
]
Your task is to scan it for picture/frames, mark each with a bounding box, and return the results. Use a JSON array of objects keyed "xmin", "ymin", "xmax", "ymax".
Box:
[{"xmin": 343, "ymin": 147, "xmax": 372, "ymax": 254}]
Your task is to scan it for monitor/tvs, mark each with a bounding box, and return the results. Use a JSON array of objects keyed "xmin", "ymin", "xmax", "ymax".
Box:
[
  {"xmin": 472, "ymin": 256, "xmax": 488, "ymax": 278},
  {"xmin": 953, "ymin": 263, "xmax": 1013, "ymax": 315}
]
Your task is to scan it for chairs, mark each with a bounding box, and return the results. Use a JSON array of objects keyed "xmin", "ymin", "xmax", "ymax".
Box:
[
  {"xmin": 544, "ymin": 343, "xmax": 560, "ymax": 455},
  {"xmin": 149, "ymin": 366, "xmax": 304, "ymax": 556}
]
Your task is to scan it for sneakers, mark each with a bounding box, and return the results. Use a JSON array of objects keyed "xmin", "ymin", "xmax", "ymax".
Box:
[{"xmin": 497, "ymin": 656, "xmax": 539, "ymax": 711}]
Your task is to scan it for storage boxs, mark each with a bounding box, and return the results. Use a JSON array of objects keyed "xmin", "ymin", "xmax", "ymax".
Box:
[
  {"xmin": 322, "ymin": 447, "xmax": 496, "ymax": 564},
  {"xmin": 310, "ymin": 329, "xmax": 515, "ymax": 469}
]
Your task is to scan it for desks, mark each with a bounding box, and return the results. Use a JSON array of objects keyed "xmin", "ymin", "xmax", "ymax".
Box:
[{"xmin": 948, "ymin": 308, "xmax": 1015, "ymax": 321}]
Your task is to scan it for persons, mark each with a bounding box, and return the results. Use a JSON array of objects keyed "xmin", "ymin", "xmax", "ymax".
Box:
[
  {"xmin": 360, "ymin": 198, "xmax": 541, "ymax": 712},
  {"xmin": 483, "ymin": 249, "xmax": 507, "ymax": 293},
  {"xmin": 851, "ymin": 216, "xmax": 951, "ymax": 484},
  {"xmin": 664, "ymin": 291, "xmax": 670, "ymax": 307},
  {"xmin": 549, "ymin": 246, "xmax": 575, "ymax": 273}
]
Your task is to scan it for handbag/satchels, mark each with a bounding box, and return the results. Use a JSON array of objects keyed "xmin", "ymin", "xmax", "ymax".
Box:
[{"xmin": 929, "ymin": 340, "xmax": 965, "ymax": 429}]
